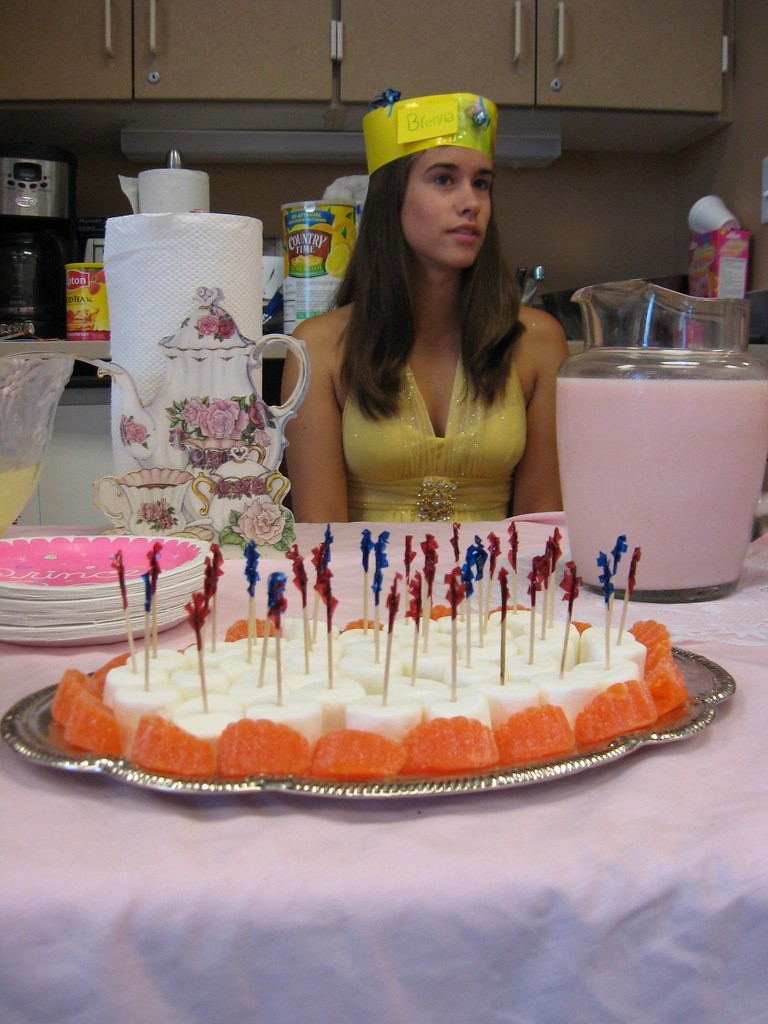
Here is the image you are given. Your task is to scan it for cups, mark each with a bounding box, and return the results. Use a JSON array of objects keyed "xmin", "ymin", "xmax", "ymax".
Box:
[
  {"xmin": 688, "ymin": 194, "xmax": 741, "ymax": 234},
  {"xmin": 0, "ymin": 344, "xmax": 75, "ymax": 532}
]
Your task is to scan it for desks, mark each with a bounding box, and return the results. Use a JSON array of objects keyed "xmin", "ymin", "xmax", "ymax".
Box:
[{"xmin": 2, "ymin": 512, "xmax": 766, "ymax": 1022}]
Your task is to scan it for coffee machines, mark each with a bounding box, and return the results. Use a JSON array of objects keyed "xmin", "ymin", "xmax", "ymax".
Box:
[{"xmin": 1, "ymin": 137, "xmax": 78, "ymax": 344}]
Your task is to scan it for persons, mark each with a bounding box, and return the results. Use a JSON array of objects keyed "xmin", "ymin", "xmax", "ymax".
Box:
[{"xmin": 281, "ymin": 92, "xmax": 570, "ymax": 523}]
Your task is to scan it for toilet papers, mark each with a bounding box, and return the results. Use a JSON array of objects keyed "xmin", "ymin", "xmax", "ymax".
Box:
[{"xmin": 102, "ymin": 163, "xmax": 268, "ymax": 479}]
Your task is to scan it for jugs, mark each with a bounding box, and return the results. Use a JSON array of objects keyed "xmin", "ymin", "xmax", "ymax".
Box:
[
  {"xmin": 78, "ymin": 286, "xmax": 315, "ymax": 560},
  {"xmin": 552, "ymin": 277, "xmax": 767, "ymax": 604}
]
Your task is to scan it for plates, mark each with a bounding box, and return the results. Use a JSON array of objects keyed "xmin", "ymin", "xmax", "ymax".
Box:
[
  {"xmin": 0, "ymin": 535, "xmax": 217, "ymax": 649},
  {"xmin": 0, "ymin": 601, "xmax": 738, "ymax": 800}
]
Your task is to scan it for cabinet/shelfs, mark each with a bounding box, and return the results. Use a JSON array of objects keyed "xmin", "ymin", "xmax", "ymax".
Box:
[
  {"xmin": 336, "ymin": 0, "xmax": 736, "ymax": 134},
  {"xmin": 1, "ymin": 0, "xmax": 334, "ymax": 118}
]
